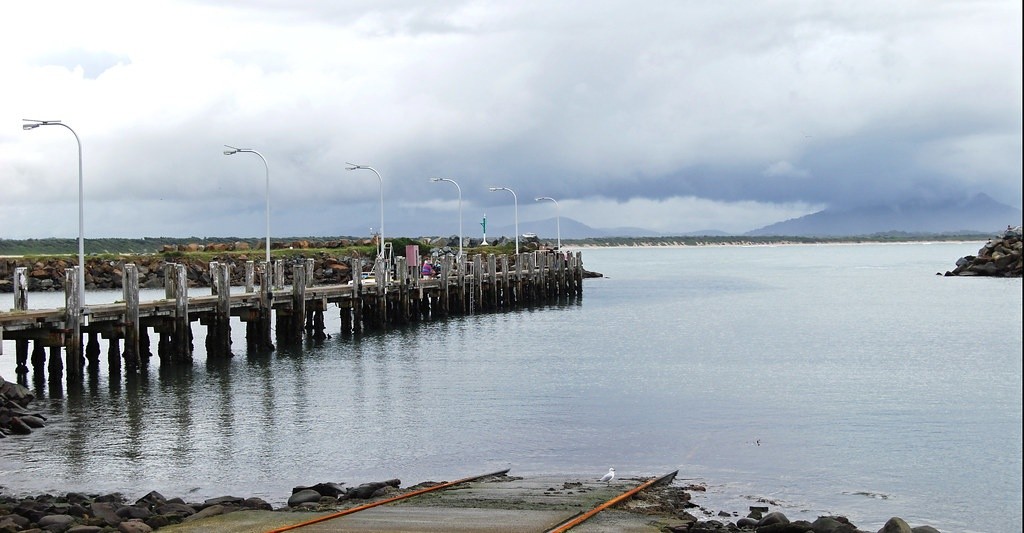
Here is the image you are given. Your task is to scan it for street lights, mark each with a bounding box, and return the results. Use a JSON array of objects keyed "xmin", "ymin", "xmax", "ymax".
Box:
[
  {"xmin": 345, "ymin": 162, "xmax": 385, "ymax": 263},
  {"xmin": 22, "ymin": 118, "xmax": 85, "ymax": 308},
  {"xmin": 223, "ymin": 144, "xmax": 271, "ymax": 264},
  {"xmin": 431, "ymin": 176, "xmax": 463, "ymax": 257},
  {"xmin": 534, "ymin": 196, "xmax": 561, "ymax": 250},
  {"xmin": 489, "ymin": 188, "xmax": 519, "ymax": 256}
]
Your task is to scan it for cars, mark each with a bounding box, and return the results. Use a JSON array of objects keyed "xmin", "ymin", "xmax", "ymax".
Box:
[{"xmin": 522, "ymin": 233, "xmax": 537, "ymax": 239}]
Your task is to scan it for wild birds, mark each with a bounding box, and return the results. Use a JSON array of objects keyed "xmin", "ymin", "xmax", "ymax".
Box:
[{"xmin": 596, "ymin": 467, "xmax": 615, "ymax": 487}]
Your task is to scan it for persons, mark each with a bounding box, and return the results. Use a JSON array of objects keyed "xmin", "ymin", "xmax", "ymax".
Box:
[
  {"xmin": 1004, "ymin": 225, "xmax": 1013, "ymax": 239},
  {"xmin": 422, "ymin": 261, "xmax": 437, "ymax": 281}
]
